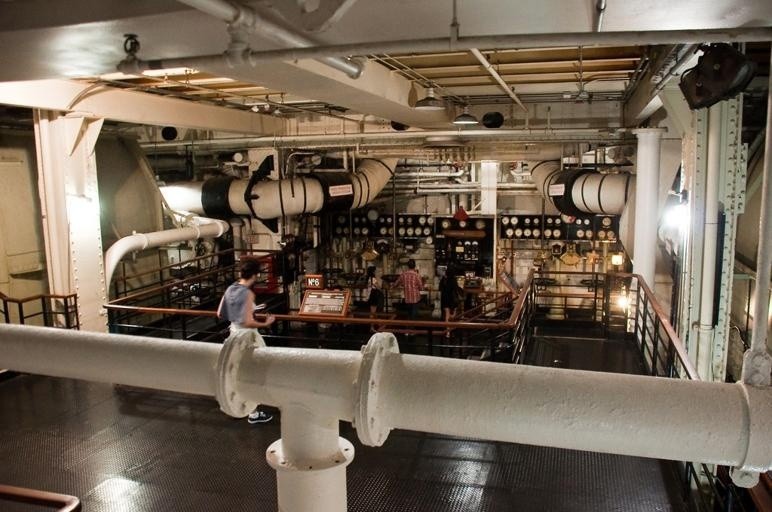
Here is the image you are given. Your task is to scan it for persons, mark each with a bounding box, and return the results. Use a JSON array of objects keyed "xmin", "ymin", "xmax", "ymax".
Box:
[
  {"xmin": 215, "ymin": 260, "xmax": 275, "ymax": 425},
  {"xmin": 364, "ymin": 266, "xmax": 382, "ymax": 335},
  {"xmin": 438, "ymin": 261, "xmax": 465, "ymax": 339},
  {"xmin": 393, "ymin": 259, "xmax": 424, "ymax": 338}
]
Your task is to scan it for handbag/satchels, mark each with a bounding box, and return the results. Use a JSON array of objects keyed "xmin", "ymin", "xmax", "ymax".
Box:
[
  {"xmin": 448, "ymin": 287, "xmax": 460, "ymax": 305},
  {"xmin": 368, "ymin": 288, "xmax": 386, "ymax": 307}
]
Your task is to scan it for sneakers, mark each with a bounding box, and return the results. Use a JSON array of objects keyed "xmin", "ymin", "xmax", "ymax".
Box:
[
  {"xmin": 247, "ymin": 412, "xmax": 273, "ymax": 424},
  {"xmin": 445, "ymin": 330, "xmax": 450, "ymax": 338},
  {"xmin": 370, "ymin": 325, "xmax": 376, "ymax": 333}
]
